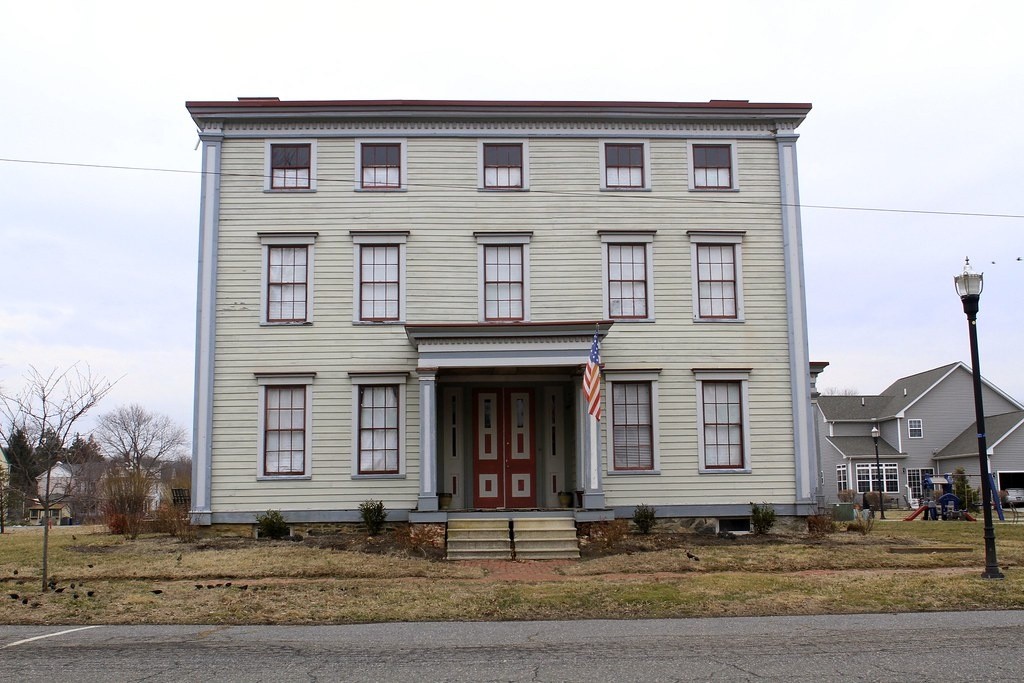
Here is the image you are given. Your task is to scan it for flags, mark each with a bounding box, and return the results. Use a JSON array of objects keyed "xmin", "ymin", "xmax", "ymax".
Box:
[{"xmin": 581, "ymin": 329, "xmax": 602, "ymax": 422}]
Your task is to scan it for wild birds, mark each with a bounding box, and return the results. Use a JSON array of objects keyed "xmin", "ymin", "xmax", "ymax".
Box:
[
  {"xmin": 194, "ymin": 582, "xmax": 232, "ymax": 591},
  {"xmin": 71, "ymin": 534, "xmax": 77, "ymax": 541},
  {"xmin": 86, "ymin": 564, "xmax": 94, "ymax": 568},
  {"xmin": 10, "ymin": 594, "xmax": 28, "ymax": 605},
  {"xmin": 150, "ymin": 590, "xmax": 163, "ymax": 595},
  {"xmin": 13, "ymin": 570, "xmax": 18, "ymax": 575},
  {"xmin": 47, "ymin": 578, "xmax": 95, "ymax": 600},
  {"xmin": 176, "ymin": 553, "xmax": 183, "ymax": 561},
  {"xmin": 687, "ymin": 553, "xmax": 700, "ymax": 561},
  {"xmin": 238, "ymin": 585, "xmax": 248, "ymax": 591}
]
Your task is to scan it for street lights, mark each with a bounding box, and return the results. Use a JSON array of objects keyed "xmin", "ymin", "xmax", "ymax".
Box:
[
  {"xmin": 953, "ymin": 257, "xmax": 1005, "ymax": 578},
  {"xmin": 872, "ymin": 425, "xmax": 888, "ymax": 520}
]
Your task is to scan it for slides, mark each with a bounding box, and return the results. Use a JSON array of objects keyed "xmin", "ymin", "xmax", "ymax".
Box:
[
  {"xmin": 963, "ymin": 512, "xmax": 977, "ymax": 521},
  {"xmin": 905, "ymin": 505, "xmax": 928, "ymax": 521}
]
